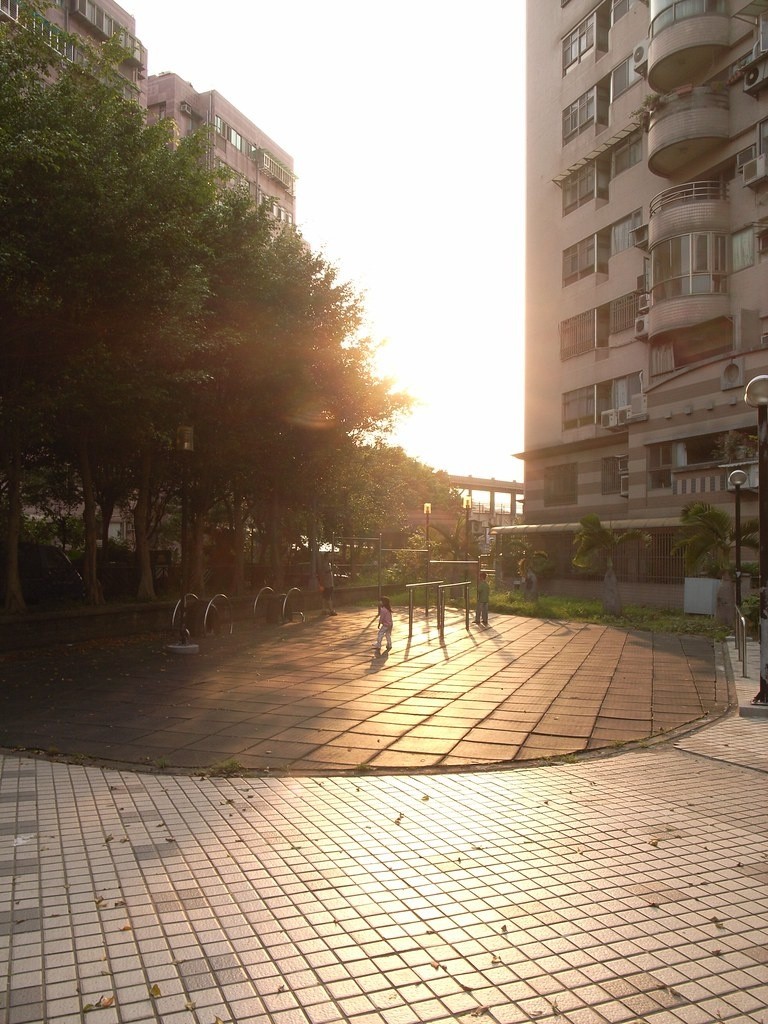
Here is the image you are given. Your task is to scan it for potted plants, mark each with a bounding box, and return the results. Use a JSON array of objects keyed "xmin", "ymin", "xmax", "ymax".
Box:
[
  {"xmin": 709, "ymin": 57, "xmax": 747, "ymax": 89},
  {"xmin": 710, "ymin": 429, "xmax": 760, "ymax": 463},
  {"xmin": 626, "ymin": 92, "xmax": 663, "ymax": 129}
]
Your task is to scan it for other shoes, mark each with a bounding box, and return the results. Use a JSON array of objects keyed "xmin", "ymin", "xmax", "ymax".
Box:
[
  {"xmin": 472, "ymin": 621, "xmax": 476, "ymax": 623},
  {"xmin": 330, "ymin": 612, "xmax": 337, "ymax": 616},
  {"xmin": 372, "ymin": 644, "xmax": 381, "ymax": 648},
  {"xmin": 385, "ymin": 647, "xmax": 392, "ymax": 652}
]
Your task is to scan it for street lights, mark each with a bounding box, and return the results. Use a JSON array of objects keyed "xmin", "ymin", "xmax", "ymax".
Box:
[
  {"xmin": 424, "ymin": 502, "xmax": 432, "ymax": 615},
  {"xmin": 730, "ymin": 470, "xmax": 748, "ymax": 608},
  {"xmin": 461, "ymin": 494, "xmax": 473, "ymax": 630},
  {"xmin": 743, "ymin": 373, "xmax": 768, "ymax": 706}
]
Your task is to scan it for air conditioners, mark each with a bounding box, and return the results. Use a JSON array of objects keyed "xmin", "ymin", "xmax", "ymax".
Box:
[
  {"xmin": 741, "ymin": 153, "xmax": 768, "ymax": 193},
  {"xmin": 744, "ymin": 57, "xmax": 768, "ymax": 95},
  {"xmin": 761, "ymin": 335, "xmax": 768, "ymax": 344},
  {"xmin": 634, "ymin": 312, "xmax": 650, "ymax": 338},
  {"xmin": 633, "ymin": 226, "xmax": 648, "ymax": 247},
  {"xmin": 631, "ymin": 392, "xmax": 648, "ymax": 417},
  {"xmin": 638, "ymin": 294, "xmax": 653, "ymax": 311},
  {"xmin": 618, "ymin": 458, "xmax": 629, "ymax": 472},
  {"xmin": 599, "ymin": 408, "xmax": 617, "ymax": 429},
  {"xmin": 632, "ymin": 34, "xmax": 648, "ymax": 77},
  {"xmin": 725, "ymin": 463, "xmax": 759, "ymax": 491},
  {"xmin": 620, "ymin": 475, "xmax": 629, "ymax": 497},
  {"xmin": 617, "ymin": 404, "xmax": 633, "ymax": 427}
]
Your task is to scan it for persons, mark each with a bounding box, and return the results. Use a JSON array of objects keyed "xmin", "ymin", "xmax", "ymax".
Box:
[
  {"xmin": 473, "ymin": 573, "xmax": 490, "ymax": 622},
  {"xmin": 318, "ymin": 552, "xmax": 338, "ymax": 616},
  {"xmin": 372, "ymin": 596, "xmax": 393, "ymax": 649}
]
